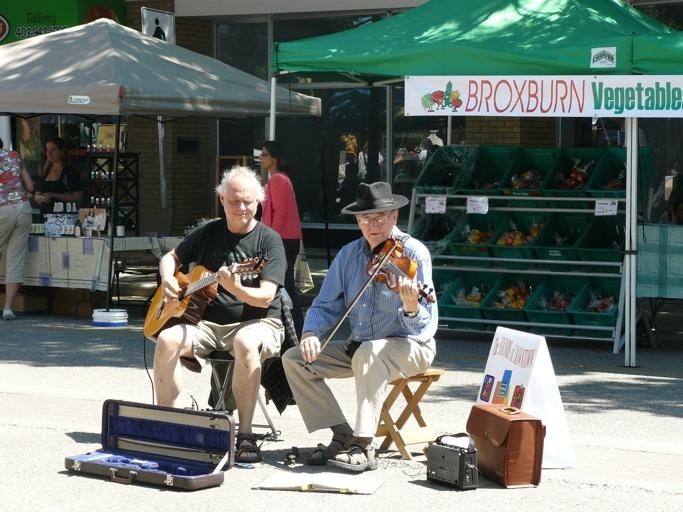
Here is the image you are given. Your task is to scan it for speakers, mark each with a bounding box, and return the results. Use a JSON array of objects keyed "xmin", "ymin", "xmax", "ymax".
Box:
[{"xmin": 427, "ymin": 441, "xmax": 478, "ymax": 490}]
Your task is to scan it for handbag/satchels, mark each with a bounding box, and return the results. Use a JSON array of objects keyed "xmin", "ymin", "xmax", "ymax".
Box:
[{"xmin": 465, "ymin": 403, "xmax": 546, "ymax": 489}]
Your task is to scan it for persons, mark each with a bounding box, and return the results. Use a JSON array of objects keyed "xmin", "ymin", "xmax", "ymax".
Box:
[
  {"xmin": 259, "ymin": 141, "xmax": 304, "ymax": 327},
  {"xmin": 0, "ymin": 138, "xmax": 33, "ymax": 319},
  {"xmin": 281, "ymin": 182, "xmax": 438, "ymax": 475},
  {"xmin": 33, "ymin": 137, "xmax": 83, "ymax": 223},
  {"xmin": 153, "ymin": 164, "xmax": 288, "ymax": 462}
]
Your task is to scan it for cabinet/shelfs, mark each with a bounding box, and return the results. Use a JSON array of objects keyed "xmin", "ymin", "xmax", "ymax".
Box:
[
  {"xmin": 405, "ymin": 144, "xmax": 652, "ymax": 355},
  {"xmin": 59, "ymin": 146, "xmax": 142, "ymax": 235}
]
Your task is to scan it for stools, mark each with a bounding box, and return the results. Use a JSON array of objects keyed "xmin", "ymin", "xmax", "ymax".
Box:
[
  {"xmin": 197, "ymin": 345, "xmax": 282, "ymax": 447},
  {"xmin": 369, "ymin": 363, "xmax": 445, "ymax": 464}
]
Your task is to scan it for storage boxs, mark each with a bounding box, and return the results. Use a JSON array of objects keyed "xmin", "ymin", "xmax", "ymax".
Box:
[
  {"xmin": 50, "ymin": 283, "xmax": 93, "ymax": 318},
  {"xmin": 0, "ymin": 291, "xmax": 49, "ymax": 316}
]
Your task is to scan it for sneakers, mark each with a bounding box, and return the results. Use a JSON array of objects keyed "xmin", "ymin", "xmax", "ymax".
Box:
[{"xmin": 2, "ymin": 309, "xmax": 16, "ymax": 320}]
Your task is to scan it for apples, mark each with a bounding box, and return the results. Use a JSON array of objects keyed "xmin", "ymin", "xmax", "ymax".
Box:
[
  {"xmin": 546, "ymin": 297, "xmax": 568, "ymax": 311},
  {"xmin": 561, "ymin": 169, "xmax": 582, "ymax": 189},
  {"xmin": 591, "ymin": 296, "xmax": 611, "ymax": 313}
]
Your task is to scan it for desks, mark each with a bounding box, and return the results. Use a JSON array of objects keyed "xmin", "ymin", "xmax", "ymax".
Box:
[
  {"xmin": 633, "ymin": 221, "xmax": 681, "ymax": 352},
  {"xmin": 0, "ymin": 228, "xmax": 189, "ymax": 320}
]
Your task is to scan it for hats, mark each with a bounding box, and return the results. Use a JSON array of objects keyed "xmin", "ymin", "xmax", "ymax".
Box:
[{"xmin": 341, "ymin": 181, "xmax": 409, "ymax": 214}]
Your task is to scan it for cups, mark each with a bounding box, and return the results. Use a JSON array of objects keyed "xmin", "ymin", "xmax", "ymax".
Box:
[
  {"xmin": 116, "ymin": 225, "xmax": 124, "ymax": 237},
  {"xmin": 479, "ymin": 374, "xmax": 494, "ymax": 402},
  {"xmin": 510, "ymin": 385, "xmax": 524, "ymax": 409},
  {"xmin": 492, "ymin": 381, "xmax": 510, "ymax": 406}
]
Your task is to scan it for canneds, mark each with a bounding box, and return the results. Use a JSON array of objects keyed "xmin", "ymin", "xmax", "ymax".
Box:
[{"xmin": 481, "ymin": 375, "xmax": 526, "ymax": 409}]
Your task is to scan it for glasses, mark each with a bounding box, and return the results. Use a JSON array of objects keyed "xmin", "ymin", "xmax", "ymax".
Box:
[{"xmin": 357, "ymin": 216, "xmax": 389, "ymax": 225}]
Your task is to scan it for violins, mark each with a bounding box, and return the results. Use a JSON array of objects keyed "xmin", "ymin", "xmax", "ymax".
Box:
[{"xmin": 367, "ymin": 238, "xmax": 435, "ymax": 308}]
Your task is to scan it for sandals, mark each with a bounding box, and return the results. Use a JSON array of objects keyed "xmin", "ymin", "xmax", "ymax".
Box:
[
  {"xmin": 236, "ymin": 433, "xmax": 261, "ymax": 462},
  {"xmin": 307, "ymin": 434, "xmax": 378, "ymax": 471}
]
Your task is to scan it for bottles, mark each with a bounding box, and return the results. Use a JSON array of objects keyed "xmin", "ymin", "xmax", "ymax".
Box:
[
  {"xmin": 90, "ymin": 172, "xmax": 113, "ymax": 180},
  {"xmin": 52, "ymin": 201, "xmax": 77, "ymax": 214},
  {"xmin": 86, "ymin": 144, "xmax": 110, "ymax": 153},
  {"xmin": 89, "ymin": 197, "xmax": 110, "ymax": 205},
  {"xmin": 59, "ymin": 222, "xmax": 80, "ymax": 236}
]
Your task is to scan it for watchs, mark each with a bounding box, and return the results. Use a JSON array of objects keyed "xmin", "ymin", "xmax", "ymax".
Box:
[{"xmin": 404, "ymin": 309, "xmax": 420, "ymax": 318}]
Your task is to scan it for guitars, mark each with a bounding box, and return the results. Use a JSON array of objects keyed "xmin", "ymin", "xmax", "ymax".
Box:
[{"xmin": 144, "ymin": 258, "xmax": 266, "ymax": 343}]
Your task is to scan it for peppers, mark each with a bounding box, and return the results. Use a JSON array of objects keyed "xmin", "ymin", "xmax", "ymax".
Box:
[
  {"xmin": 493, "ymin": 278, "xmax": 526, "ymax": 311},
  {"xmin": 496, "ymin": 225, "xmax": 543, "ymax": 247},
  {"xmin": 465, "ymin": 228, "xmax": 492, "ymax": 254}
]
